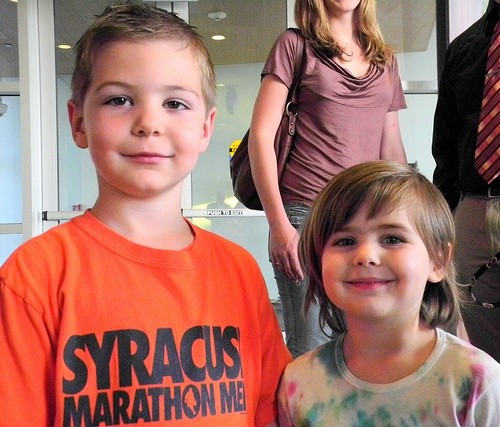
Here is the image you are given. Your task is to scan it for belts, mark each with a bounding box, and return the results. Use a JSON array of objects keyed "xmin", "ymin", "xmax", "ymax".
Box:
[{"xmin": 461, "ymin": 182, "xmax": 500, "ymax": 198}]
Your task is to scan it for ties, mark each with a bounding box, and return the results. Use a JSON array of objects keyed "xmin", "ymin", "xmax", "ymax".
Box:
[{"xmin": 474, "ymin": 20, "xmax": 500, "ymax": 184}]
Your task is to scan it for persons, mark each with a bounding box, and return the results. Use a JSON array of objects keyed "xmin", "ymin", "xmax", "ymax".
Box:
[
  {"xmin": 431, "ymin": 0, "xmax": 500, "ymax": 361},
  {"xmin": 276, "ymin": 160, "xmax": 500, "ymax": 427},
  {"xmin": 248, "ymin": 0, "xmax": 407, "ymax": 359},
  {"xmin": 0, "ymin": 0, "xmax": 292, "ymax": 427}
]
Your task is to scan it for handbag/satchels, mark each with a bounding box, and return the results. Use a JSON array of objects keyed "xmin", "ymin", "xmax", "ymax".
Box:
[{"xmin": 230, "ymin": 28, "xmax": 306, "ymax": 209}]
